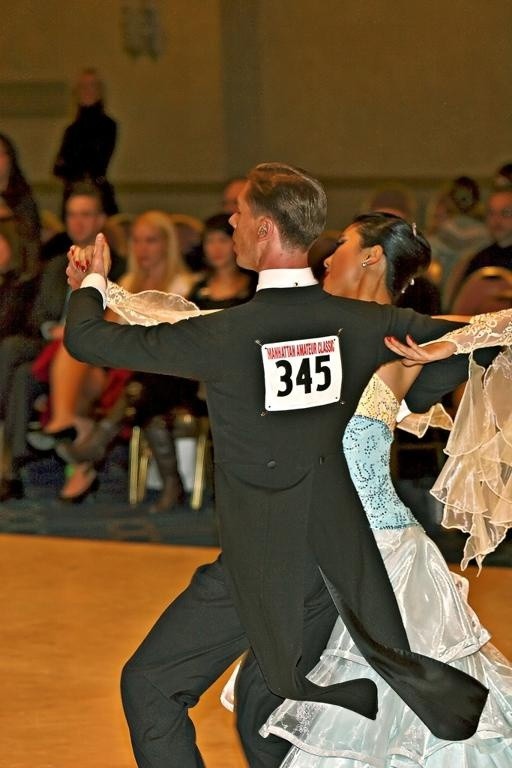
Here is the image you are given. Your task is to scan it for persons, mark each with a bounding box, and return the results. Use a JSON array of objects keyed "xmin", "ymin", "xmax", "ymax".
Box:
[
  {"xmin": 2, "ymin": 133, "xmax": 258, "ymax": 517},
  {"xmin": 316, "ymin": 161, "xmax": 511, "ymax": 408},
  {"xmin": 50, "ymin": 68, "xmax": 124, "ymax": 224}
]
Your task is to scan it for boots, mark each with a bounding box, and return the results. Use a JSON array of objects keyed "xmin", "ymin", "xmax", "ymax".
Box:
[
  {"xmin": 59, "ymin": 415, "xmax": 123, "ymax": 477},
  {"xmin": 149, "ymin": 455, "xmax": 188, "ymax": 518}
]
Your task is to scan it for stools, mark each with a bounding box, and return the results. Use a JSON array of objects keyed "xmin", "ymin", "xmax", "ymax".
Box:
[
  {"xmin": 127, "ymin": 415, "xmax": 212, "ymax": 509},
  {"xmin": 389, "ymin": 432, "xmax": 446, "ymax": 482}
]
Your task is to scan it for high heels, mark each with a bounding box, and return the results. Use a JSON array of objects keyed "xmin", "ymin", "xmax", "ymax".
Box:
[
  {"xmin": 24, "ymin": 422, "xmax": 78, "ymax": 457},
  {"xmin": 53, "ymin": 473, "xmax": 103, "ymax": 510}
]
